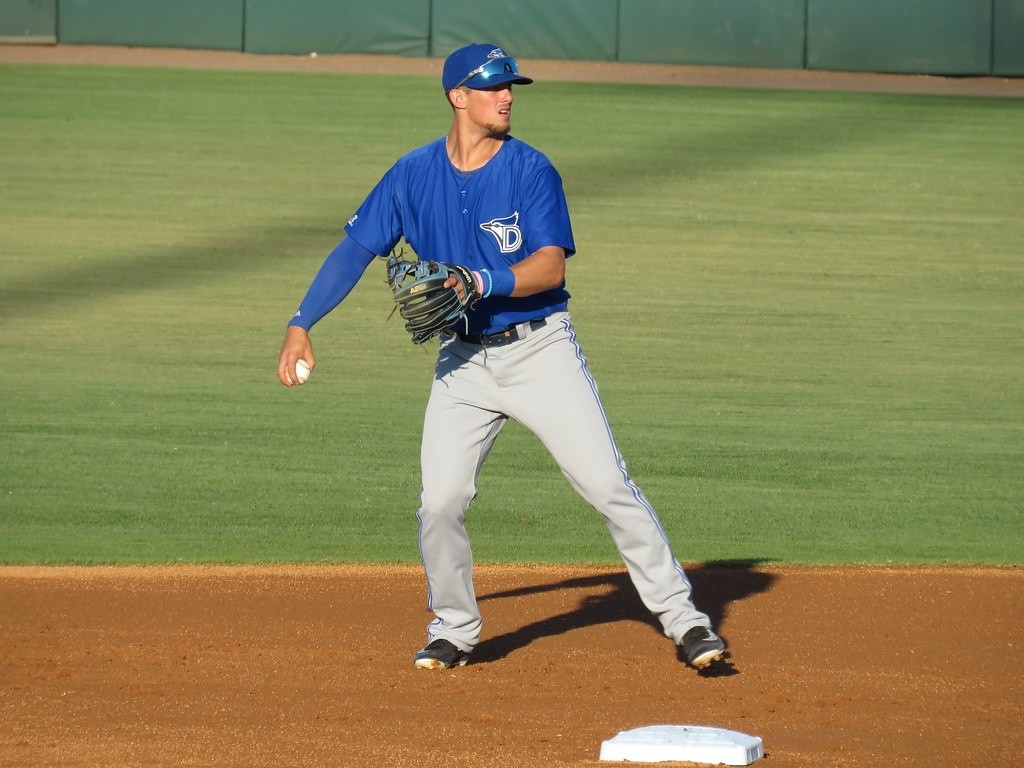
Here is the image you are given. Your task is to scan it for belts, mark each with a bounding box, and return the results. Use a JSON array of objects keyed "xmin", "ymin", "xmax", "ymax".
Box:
[{"xmin": 460, "ymin": 317, "xmax": 547, "ymax": 346}]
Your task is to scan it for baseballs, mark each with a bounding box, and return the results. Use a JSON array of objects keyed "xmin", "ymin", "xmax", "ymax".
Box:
[{"xmin": 285, "ymin": 359, "xmax": 311, "ymax": 385}]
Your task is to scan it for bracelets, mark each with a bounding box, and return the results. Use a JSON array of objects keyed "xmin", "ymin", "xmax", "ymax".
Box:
[
  {"xmin": 479, "ymin": 269, "xmax": 516, "ymax": 297},
  {"xmin": 472, "ymin": 271, "xmax": 483, "ymax": 294},
  {"xmin": 480, "ymin": 269, "xmax": 492, "ymax": 298}
]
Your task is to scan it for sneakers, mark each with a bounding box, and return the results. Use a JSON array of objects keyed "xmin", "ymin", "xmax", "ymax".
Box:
[
  {"xmin": 414, "ymin": 639, "xmax": 471, "ymax": 669},
  {"xmin": 680, "ymin": 626, "xmax": 726, "ymax": 669}
]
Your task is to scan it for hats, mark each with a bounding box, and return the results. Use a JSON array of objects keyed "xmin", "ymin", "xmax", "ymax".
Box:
[{"xmin": 442, "ymin": 43, "xmax": 533, "ymax": 88}]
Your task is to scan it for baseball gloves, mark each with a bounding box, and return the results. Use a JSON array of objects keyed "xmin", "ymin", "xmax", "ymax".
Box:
[{"xmin": 386, "ymin": 257, "xmax": 477, "ymax": 345}]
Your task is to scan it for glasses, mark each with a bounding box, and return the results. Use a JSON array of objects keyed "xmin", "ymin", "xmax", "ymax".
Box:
[{"xmin": 453, "ymin": 57, "xmax": 518, "ymax": 88}]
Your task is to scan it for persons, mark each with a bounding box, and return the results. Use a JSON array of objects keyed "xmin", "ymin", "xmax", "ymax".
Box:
[{"xmin": 278, "ymin": 42, "xmax": 727, "ymax": 670}]
